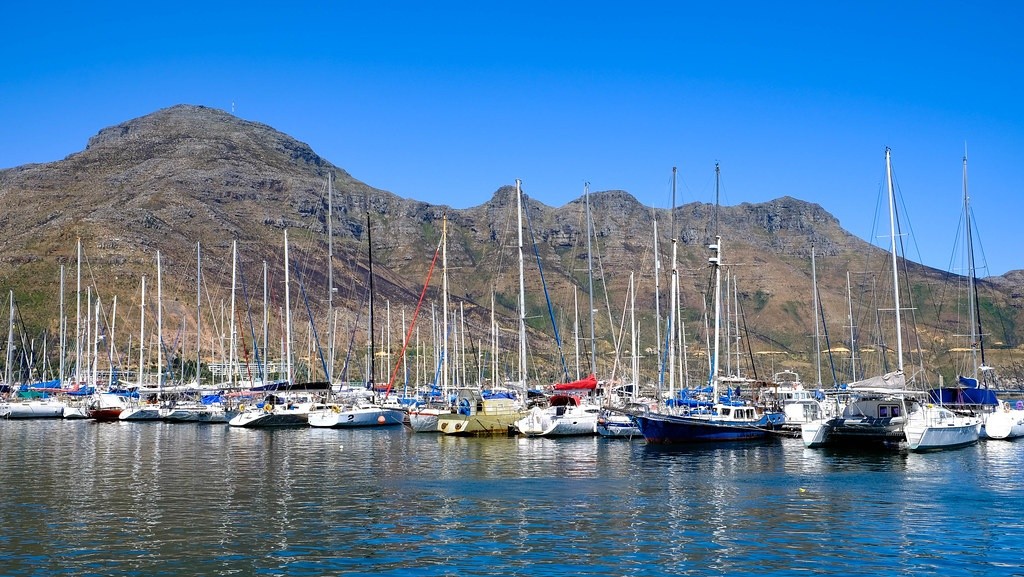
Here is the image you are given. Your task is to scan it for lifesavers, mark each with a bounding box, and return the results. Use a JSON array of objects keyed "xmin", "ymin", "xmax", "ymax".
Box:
[
  {"xmin": 440, "ymin": 423, "xmax": 446, "ymax": 429},
  {"xmin": 1004, "ymin": 402, "xmax": 1010, "ymax": 412},
  {"xmin": 239, "ymin": 404, "xmax": 245, "ymax": 411},
  {"xmin": 264, "ymin": 405, "xmax": 271, "ymax": 412},
  {"xmin": 455, "ymin": 423, "xmax": 461, "ymax": 430},
  {"xmin": 1016, "ymin": 402, "xmax": 1023, "ymax": 409}
]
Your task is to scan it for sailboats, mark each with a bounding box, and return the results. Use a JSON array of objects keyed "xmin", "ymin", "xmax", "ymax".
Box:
[{"xmin": 0, "ymin": 147, "xmax": 1024, "ymax": 455}]
